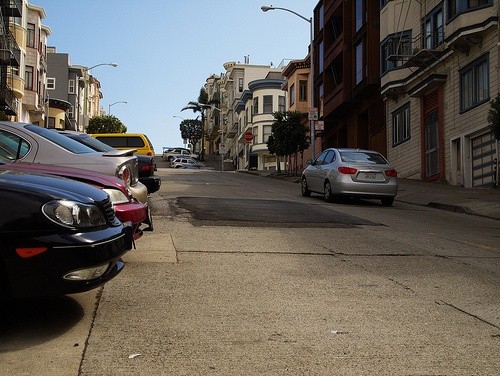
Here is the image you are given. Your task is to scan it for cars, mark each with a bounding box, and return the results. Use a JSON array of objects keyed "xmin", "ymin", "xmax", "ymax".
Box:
[
  {"xmin": 0, "ymin": 120, "xmax": 152, "ymax": 206},
  {"xmin": 162, "ymin": 147, "xmax": 206, "ymax": 171},
  {"xmin": 300, "ymin": 148, "xmax": 399, "ymax": 206},
  {"xmin": 0, "ymin": 153, "xmax": 154, "ymax": 243},
  {"xmin": 0, "ymin": 166, "xmax": 133, "ymax": 326},
  {"xmin": 33, "ymin": 129, "xmax": 162, "ymax": 195}
]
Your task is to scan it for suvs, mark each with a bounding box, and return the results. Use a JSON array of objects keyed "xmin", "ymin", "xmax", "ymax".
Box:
[{"xmin": 88, "ymin": 133, "xmax": 156, "ymax": 162}]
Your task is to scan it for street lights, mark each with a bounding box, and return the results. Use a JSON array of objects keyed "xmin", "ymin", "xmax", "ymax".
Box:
[
  {"xmin": 199, "ymin": 102, "xmax": 224, "ymax": 171},
  {"xmin": 84, "ymin": 63, "xmax": 118, "ymax": 133},
  {"xmin": 108, "ymin": 101, "xmax": 128, "ymax": 116},
  {"xmin": 261, "ymin": 4, "xmax": 315, "ymax": 162},
  {"xmin": 174, "ymin": 115, "xmax": 192, "ymax": 151}
]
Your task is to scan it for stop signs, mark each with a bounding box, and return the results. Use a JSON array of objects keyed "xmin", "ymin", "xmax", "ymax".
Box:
[{"xmin": 244, "ymin": 131, "xmax": 254, "ymax": 142}]
